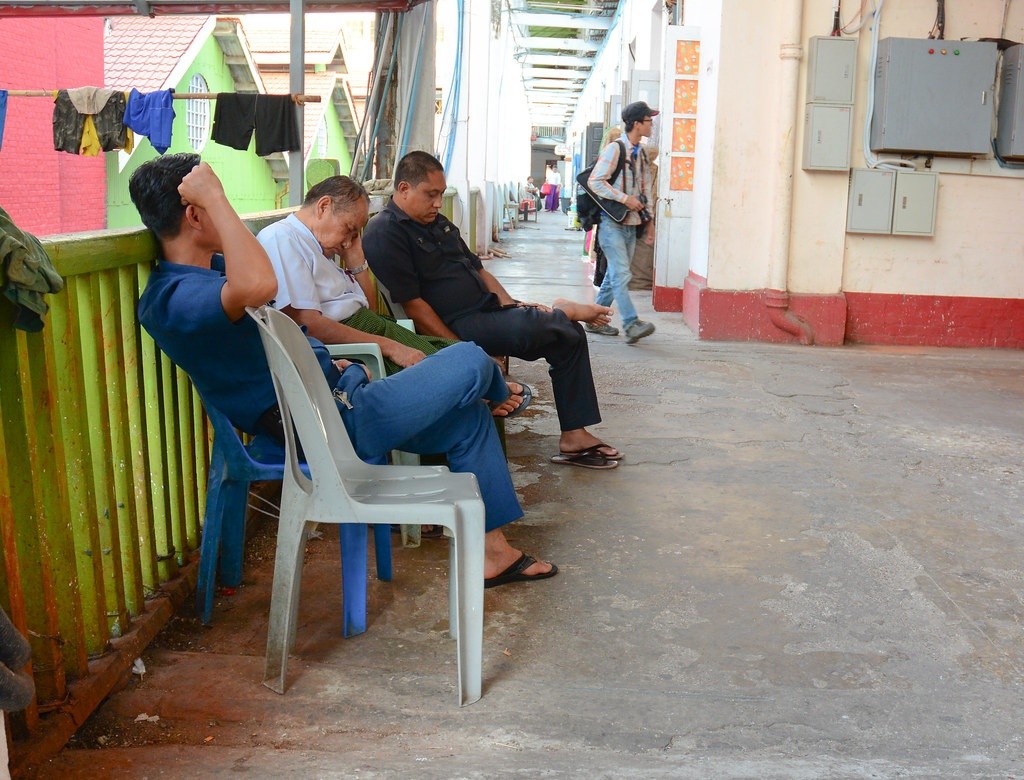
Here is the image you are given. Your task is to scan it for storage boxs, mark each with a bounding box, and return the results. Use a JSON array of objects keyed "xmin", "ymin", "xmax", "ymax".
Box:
[{"xmin": 567, "ymin": 210, "xmax": 578, "ymax": 229}]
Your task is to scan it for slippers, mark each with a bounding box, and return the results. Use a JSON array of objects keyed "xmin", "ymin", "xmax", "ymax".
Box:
[
  {"xmin": 391, "ymin": 521, "xmax": 441, "ymax": 537},
  {"xmin": 485, "ymin": 550, "xmax": 558, "ymax": 589},
  {"xmin": 550, "ymin": 449, "xmax": 618, "ymax": 469},
  {"xmin": 490, "ymin": 381, "xmax": 531, "ymax": 419},
  {"xmin": 559, "ymin": 443, "xmax": 624, "ymax": 459}
]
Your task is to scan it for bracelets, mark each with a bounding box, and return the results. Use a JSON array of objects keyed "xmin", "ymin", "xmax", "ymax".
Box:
[{"xmin": 345, "ymin": 259, "xmax": 368, "ymax": 274}]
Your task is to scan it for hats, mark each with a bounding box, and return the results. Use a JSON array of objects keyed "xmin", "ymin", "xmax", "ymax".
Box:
[{"xmin": 621, "ymin": 101, "xmax": 659, "ymax": 122}]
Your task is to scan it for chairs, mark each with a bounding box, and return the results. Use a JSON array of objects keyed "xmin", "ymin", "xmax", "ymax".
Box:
[
  {"xmin": 321, "ymin": 317, "xmax": 427, "ymax": 551},
  {"xmin": 187, "ymin": 373, "xmax": 394, "ymax": 639},
  {"xmin": 245, "ymin": 305, "xmax": 487, "ymax": 708},
  {"xmin": 510, "ymin": 190, "xmax": 538, "ymax": 223}
]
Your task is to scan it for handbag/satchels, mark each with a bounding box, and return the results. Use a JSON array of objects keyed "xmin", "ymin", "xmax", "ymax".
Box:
[
  {"xmin": 541, "ymin": 183, "xmax": 550, "ymax": 194},
  {"xmin": 575, "ymin": 140, "xmax": 630, "ymax": 223}
]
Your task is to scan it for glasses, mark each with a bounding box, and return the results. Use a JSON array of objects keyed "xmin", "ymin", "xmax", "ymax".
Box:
[{"xmin": 640, "ymin": 119, "xmax": 653, "ymax": 125}]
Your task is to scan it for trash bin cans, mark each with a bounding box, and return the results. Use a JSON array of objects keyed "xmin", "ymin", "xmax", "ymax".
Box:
[{"xmin": 561, "ymin": 197, "xmax": 571, "ymax": 213}]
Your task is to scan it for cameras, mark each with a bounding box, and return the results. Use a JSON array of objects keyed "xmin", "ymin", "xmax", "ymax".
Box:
[{"xmin": 636, "ymin": 194, "xmax": 651, "ymax": 224}]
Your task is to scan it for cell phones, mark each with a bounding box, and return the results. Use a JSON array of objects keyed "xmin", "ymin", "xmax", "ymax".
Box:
[{"xmin": 483, "ymin": 304, "xmax": 517, "ymax": 313}]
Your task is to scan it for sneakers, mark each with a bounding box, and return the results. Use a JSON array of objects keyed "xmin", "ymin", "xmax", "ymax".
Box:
[
  {"xmin": 584, "ymin": 322, "xmax": 619, "ymax": 335},
  {"xmin": 624, "ymin": 320, "xmax": 655, "ymax": 343}
]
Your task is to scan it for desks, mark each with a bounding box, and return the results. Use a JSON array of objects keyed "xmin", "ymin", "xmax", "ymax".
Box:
[{"xmin": 503, "ymin": 203, "xmax": 519, "ymax": 230}]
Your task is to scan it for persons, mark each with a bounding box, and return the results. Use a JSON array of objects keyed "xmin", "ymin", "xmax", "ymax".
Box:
[
  {"xmin": 362, "ymin": 150, "xmax": 625, "ymax": 470},
  {"xmin": 128, "ymin": 153, "xmax": 557, "ymax": 588},
  {"xmin": 524, "ymin": 176, "xmax": 542, "ymax": 211},
  {"xmin": 584, "ymin": 101, "xmax": 659, "ymax": 344},
  {"xmin": 544, "ymin": 163, "xmax": 561, "ymax": 213},
  {"xmin": 256, "ymin": 175, "xmax": 507, "ymax": 538}
]
